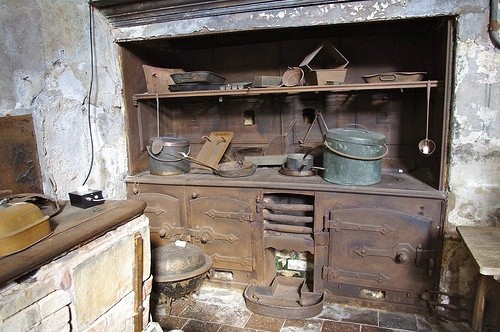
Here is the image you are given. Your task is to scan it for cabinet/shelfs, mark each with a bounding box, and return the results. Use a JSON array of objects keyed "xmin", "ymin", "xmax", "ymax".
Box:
[{"xmin": 120, "ymin": 19, "xmax": 453, "ymax": 314}]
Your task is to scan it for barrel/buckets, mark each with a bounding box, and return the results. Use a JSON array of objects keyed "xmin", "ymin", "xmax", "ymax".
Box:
[
  {"xmin": 323, "ymin": 124, "xmax": 389, "ymax": 185},
  {"xmin": 146, "ymin": 135, "xmax": 191, "ymax": 175}
]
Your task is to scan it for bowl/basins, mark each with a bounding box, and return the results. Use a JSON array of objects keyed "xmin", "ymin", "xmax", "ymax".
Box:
[{"xmin": 281, "ymin": 67, "xmax": 305, "ymax": 86}]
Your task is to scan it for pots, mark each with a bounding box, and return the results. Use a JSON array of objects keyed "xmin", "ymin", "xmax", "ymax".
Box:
[
  {"xmin": 217, "ymin": 160, "xmax": 258, "ymax": 177},
  {"xmin": 287, "ymin": 153, "xmax": 325, "ymax": 171},
  {"xmin": 151, "ymin": 242, "xmax": 213, "ymax": 298}
]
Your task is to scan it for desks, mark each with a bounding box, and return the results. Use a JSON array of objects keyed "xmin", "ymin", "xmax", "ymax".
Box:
[{"xmin": 456, "ymin": 225, "xmax": 500, "ymax": 332}]
